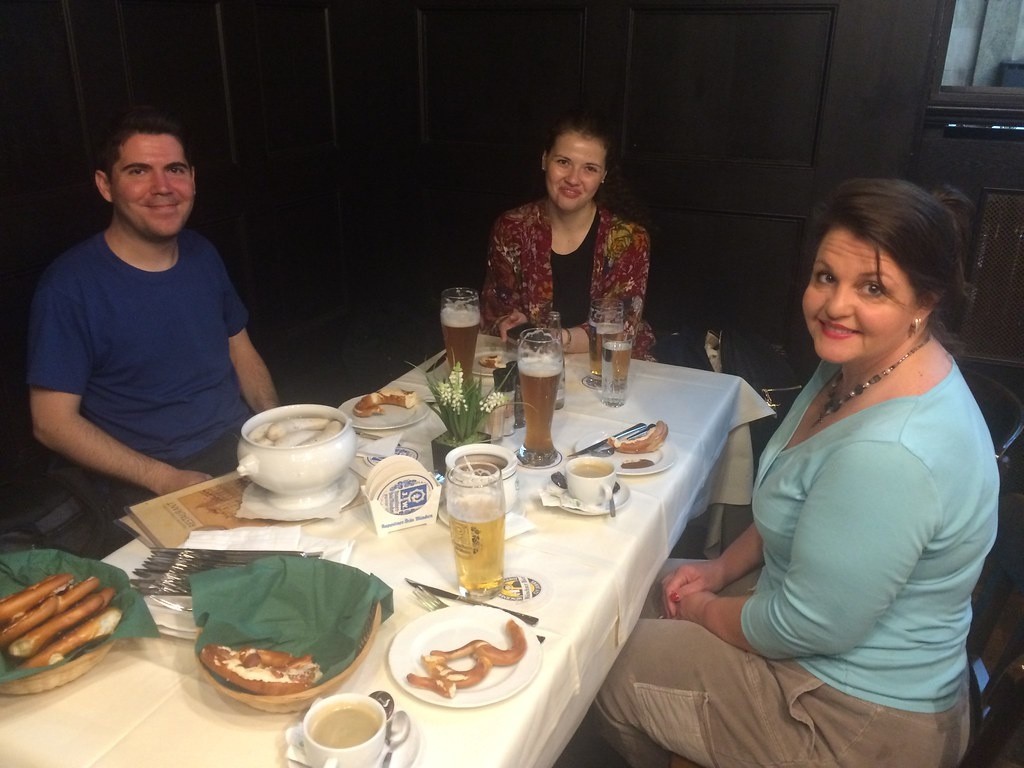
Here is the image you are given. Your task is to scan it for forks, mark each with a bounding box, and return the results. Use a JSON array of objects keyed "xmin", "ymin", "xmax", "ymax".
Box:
[
  {"xmin": 567, "ymin": 423, "xmax": 647, "ymax": 457},
  {"xmin": 589, "ymin": 424, "xmax": 656, "ymax": 457},
  {"xmin": 412, "ymin": 584, "xmax": 449, "ymax": 612}
]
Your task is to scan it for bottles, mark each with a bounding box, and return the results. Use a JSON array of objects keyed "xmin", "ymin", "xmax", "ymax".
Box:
[{"xmin": 546, "ymin": 311, "xmax": 565, "ymax": 410}]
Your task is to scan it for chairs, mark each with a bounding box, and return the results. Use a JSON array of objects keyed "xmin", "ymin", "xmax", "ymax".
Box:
[
  {"xmin": 963, "ymin": 365, "xmax": 1024, "ymax": 481},
  {"xmin": 668, "ymin": 492, "xmax": 1024, "ymax": 768}
]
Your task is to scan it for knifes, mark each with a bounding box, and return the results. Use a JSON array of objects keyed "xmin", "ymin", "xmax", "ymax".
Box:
[
  {"xmin": 425, "ymin": 351, "xmax": 447, "ymax": 374},
  {"xmin": 405, "ymin": 577, "xmax": 539, "ymax": 626},
  {"xmin": 130, "ymin": 546, "xmax": 324, "ymax": 597}
]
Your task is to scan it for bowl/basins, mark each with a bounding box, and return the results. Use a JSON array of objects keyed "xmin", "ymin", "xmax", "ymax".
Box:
[
  {"xmin": 194, "ymin": 600, "xmax": 382, "ymax": 715},
  {"xmin": 236, "ymin": 404, "xmax": 358, "ymax": 511}
]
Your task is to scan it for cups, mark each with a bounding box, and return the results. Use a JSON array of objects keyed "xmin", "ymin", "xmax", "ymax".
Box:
[
  {"xmin": 601, "ymin": 331, "xmax": 633, "ymax": 408},
  {"xmin": 301, "ymin": 693, "xmax": 387, "ymax": 768},
  {"xmin": 445, "ymin": 462, "xmax": 507, "ymax": 604},
  {"xmin": 513, "ymin": 328, "xmax": 563, "ymax": 470},
  {"xmin": 565, "ymin": 456, "xmax": 617, "ymax": 506},
  {"xmin": 580, "ymin": 298, "xmax": 624, "ymax": 390},
  {"xmin": 439, "ymin": 287, "xmax": 481, "ymax": 381},
  {"xmin": 444, "ymin": 443, "xmax": 518, "ymax": 515}
]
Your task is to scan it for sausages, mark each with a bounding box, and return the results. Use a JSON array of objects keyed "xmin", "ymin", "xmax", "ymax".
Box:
[{"xmin": 247, "ymin": 416, "xmax": 344, "ymax": 447}]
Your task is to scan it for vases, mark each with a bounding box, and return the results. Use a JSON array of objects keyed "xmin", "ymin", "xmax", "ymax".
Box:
[{"xmin": 431, "ymin": 428, "xmax": 493, "ymax": 477}]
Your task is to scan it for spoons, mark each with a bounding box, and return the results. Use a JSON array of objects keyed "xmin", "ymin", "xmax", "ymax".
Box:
[
  {"xmin": 369, "ymin": 690, "xmax": 394, "ymax": 720},
  {"xmin": 380, "ymin": 710, "xmax": 411, "ymax": 768}
]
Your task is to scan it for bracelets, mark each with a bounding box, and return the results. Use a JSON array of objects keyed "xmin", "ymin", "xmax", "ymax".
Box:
[{"xmin": 563, "ymin": 327, "xmax": 572, "ymax": 347}]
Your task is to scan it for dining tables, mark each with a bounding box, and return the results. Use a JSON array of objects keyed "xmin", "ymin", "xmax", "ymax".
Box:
[{"xmin": 0, "ymin": 333, "xmax": 778, "ymax": 768}]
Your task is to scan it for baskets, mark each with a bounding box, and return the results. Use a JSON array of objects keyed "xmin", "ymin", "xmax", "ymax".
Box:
[
  {"xmin": 195, "ymin": 598, "xmax": 382, "ymax": 715},
  {"xmin": 0, "ymin": 549, "xmax": 128, "ymax": 695}
]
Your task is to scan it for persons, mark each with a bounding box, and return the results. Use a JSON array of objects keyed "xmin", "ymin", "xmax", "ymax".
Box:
[
  {"xmin": 476, "ymin": 111, "xmax": 656, "ymax": 362},
  {"xmin": 593, "ymin": 173, "xmax": 1004, "ymax": 768},
  {"xmin": 26, "ymin": 104, "xmax": 279, "ymax": 556}
]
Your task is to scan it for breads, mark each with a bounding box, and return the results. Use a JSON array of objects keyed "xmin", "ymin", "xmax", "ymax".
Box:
[
  {"xmin": 606, "ymin": 421, "xmax": 669, "ymax": 454},
  {"xmin": 200, "ymin": 643, "xmax": 323, "ymax": 696},
  {"xmin": 479, "ymin": 355, "xmax": 506, "ymax": 368},
  {"xmin": 353, "ymin": 388, "xmax": 416, "ymax": 417},
  {"xmin": 406, "ymin": 618, "xmax": 528, "ymax": 698},
  {"xmin": 0, "ymin": 572, "xmax": 122, "ymax": 669}
]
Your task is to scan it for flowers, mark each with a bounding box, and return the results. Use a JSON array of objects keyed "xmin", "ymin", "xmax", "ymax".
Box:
[{"xmin": 401, "ymin": 341, "xmax": 541, "ymax": 441}]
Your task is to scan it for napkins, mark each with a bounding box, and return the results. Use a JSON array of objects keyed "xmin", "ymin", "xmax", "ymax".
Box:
[
  {"xmin": 505, "ymin": 511, "xmax": 535, "ymax": 541},
  {"xmin": 356, "ymin": 431, "xmax": 404, "ymax": 460},
  {"xmin": 155, "ymin": 523, "xmax": 301, "ymax": 607}
]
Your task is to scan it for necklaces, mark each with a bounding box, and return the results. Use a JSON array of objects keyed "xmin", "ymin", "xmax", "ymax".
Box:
[{"xmin": 809, "ymin": 333, "xmax": 931, "ymax": 427}]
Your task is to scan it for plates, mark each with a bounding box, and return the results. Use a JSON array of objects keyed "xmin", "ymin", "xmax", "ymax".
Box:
[
  {"xmin": 337, "ymin": 395, "xmax": 430, "ymax": 431},
  {"xmin": 472, "ymin": 346, "xmax": 518, "ymax": 377},
  {"xmin": 287, "ymin": 707, "xmax": 423, "ymax": 768},
  {"xmin": 241, "ymin": 472, "xmax": 360, "ymax": 522},
  {"xmin": 143, "ymin": 596, "xmax": 196, "ymax": 641},
  {"xmin": 438, "ymin": 492, "xmax": 526, "ymax": 529},
  {"xmin": 575, "ymin": 425, "xmax": 680, "ymax": 476},
  {"xmin": 388, "ymin": 605, "xmax": 542, "ymax": 708},
  {"xmin": 560, "ymin": 477, "xmax": 631, "ymax": 517}
]
